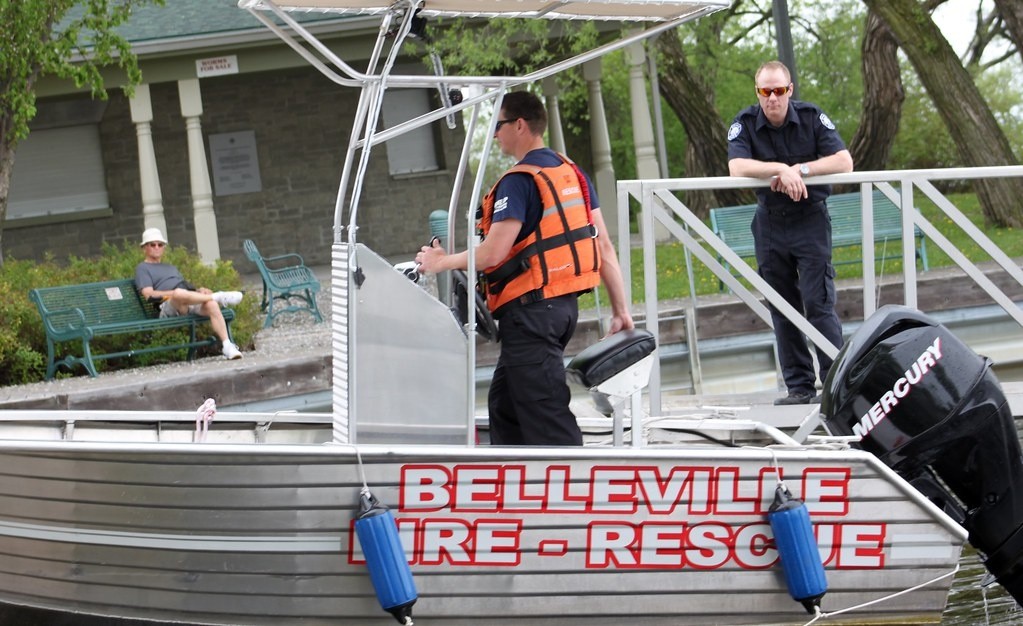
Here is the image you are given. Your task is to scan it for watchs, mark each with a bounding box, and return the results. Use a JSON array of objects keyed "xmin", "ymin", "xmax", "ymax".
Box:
[{"xmin": 799, "ymin": 163, "xmax": 810, "ymax": 177}]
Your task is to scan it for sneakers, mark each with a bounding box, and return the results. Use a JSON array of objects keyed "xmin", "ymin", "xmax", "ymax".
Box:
[
  {"xmin": 221, "ymin": 343, "xmax": 243, "ymax": 360},
  {"xmin": 218, "ymin": 291, "xmax": 242, "ymax": 308}
]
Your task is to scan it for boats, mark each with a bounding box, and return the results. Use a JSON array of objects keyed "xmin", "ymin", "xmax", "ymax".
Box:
[{"xmin": 0, "ymin": 0, "xmax": 1023, "ymax": 626}]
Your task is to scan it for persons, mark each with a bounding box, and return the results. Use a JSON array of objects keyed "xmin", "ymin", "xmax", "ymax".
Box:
[
  {"xmin": 414, "ymin": 90, "xmax": 635, "ymax": 446},
  {"xmin": 726, "ymin": 60, "xmax": 853, "ymax": 406},
  {"xmin": 134, "ymin": 227, "xmax": 243, "ymax": 360}
]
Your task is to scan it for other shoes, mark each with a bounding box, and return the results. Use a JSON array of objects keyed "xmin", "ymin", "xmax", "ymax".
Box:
[
  {"xmin": 774, "ymin": 392, "xmax": 811, "ymax": 405},
  {"xmin": 809, "ymin": 394, "xmax": 822, "ymax": 403}
]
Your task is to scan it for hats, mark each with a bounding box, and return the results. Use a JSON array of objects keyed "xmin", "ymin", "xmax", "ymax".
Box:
[{"xmin": 140, "ymin": 228, "xmax": 167, "ymax": 246}]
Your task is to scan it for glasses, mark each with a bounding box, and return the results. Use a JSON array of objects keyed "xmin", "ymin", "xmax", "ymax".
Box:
[
  {"xmin": 757, "ymin": 83, "xmax": 791, "ymax": 97},
  {"xmin": 496, "ymin": 117, "xmax": 531, "ymax": 132},
  {"xmin": 148, "ymin": 243, "xmax": 164, "ymax": 247}
]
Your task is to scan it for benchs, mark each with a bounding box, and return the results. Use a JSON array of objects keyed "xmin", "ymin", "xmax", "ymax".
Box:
[
  {"xmin": 29, "ymin": 278, "xmax": 237, "ymax": 383},
  {"xmin": 243, "ymin": 240, "xmax": 323, "ymax": 329},
  {"xmin": 710, "ymin": 188, "xmax": 929, "ymax": 293}
]
